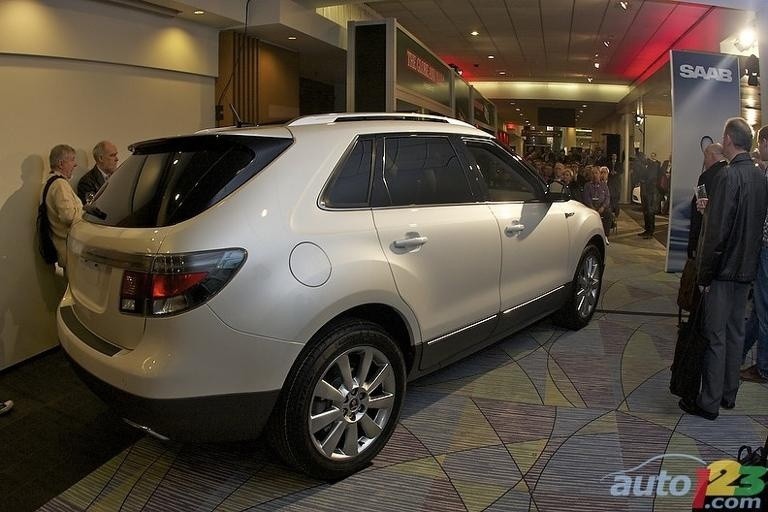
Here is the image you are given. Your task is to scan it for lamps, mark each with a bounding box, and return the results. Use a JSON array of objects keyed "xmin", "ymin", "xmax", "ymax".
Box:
[{"xmin": 734, "ymin": 26, "xmax": 756, "ymax": 52}]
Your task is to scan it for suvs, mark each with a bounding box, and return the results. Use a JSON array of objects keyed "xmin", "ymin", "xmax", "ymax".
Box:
[{"xmin": 52, "ymin": 109, "xmax": 611, "ymax": 478}]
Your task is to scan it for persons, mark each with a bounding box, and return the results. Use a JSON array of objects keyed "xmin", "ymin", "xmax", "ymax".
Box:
[
  {"xmin": 523, "ymin": 142, "xmax": 670, "ymax": 246},
  {"xmin": 76, "ymin": 141, "xmax": 118, "ymax": 207},
  {"xmin": 38, "ymin": 144, "xmax": 85, "ymax": 281},
  {"xmin": 674, "ymin": 115, "xmax": 767, "ymax": 421}
]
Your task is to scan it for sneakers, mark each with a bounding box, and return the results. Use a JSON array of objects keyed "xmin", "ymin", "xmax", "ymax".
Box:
[
  {"xmin": 638, "ymin": 229, "xmax": 653, "ymax": 240},
  {"xmin": 720, "ymin": 398, "xmax": 737, "ymax": 411},
  {"xmin": 0, "ymin": 399, "xmax": 15, "ymax": 413}
]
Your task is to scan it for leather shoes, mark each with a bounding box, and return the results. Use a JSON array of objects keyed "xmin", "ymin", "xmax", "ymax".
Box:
[
  {"xmin": 677, "ymin": 394, "xmax": 720, "ymax": 422},
  {"xmin": 737, "ymin": 362, "xmax": 767, "ymax": 383}
]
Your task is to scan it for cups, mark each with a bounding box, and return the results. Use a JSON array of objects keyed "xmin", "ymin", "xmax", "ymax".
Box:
[
  {"xmin": 693, "ymin": 184, "xmax": 708, "ymax": 215},
  {"xmin": 84, "ymin": 191, "xmax": 95, "ymax": 205}
]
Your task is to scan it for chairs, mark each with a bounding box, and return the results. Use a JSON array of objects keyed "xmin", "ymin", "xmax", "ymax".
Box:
[{"xmin": 333, "ymin": 164, "xmax": 468, "ymax": 205}]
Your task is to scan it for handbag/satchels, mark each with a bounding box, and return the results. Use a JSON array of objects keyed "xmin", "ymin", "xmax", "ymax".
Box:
[{"xmin": 33, "ymin": 175, "xmax": 70, "ymax": 265}]
